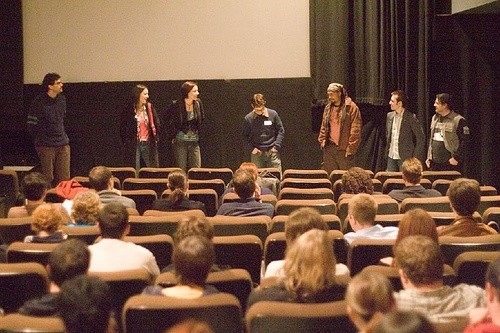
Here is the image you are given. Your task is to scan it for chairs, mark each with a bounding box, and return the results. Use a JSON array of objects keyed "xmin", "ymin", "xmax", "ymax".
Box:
[{"xmin": 0, "ymin": 167, "xmax": 500, "ymax": 333}]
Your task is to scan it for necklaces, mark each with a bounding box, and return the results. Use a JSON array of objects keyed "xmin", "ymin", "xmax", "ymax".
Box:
[{"xmin": 186, "ymin": 102, "xmax": 194, "ymax": 111}]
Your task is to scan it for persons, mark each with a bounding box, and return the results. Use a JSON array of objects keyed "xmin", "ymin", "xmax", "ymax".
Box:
[
  {"xmin": 385, "ymin": 91, "xmax": 426, "ymax": 172},
  {"xmin": 119, "ymin": 84, "xmax": 159, "ymax": 170},
  {"xmin": 165, "ymin": 82, "xmax": 204, "ymax": 170},
  {"xmin": 0, "ymin": 158, "xmax": 500, "ymax": 333},
  {"xmin": 318, "ymin": 83, "xmax": 362, "ymax": 172},
  {"xmin": 426, "ymin": 93, "xmax": 471, "ymax": 175},
  {"xmin": 243, "ymin": 94, "xmax": 284, "ymax": 180},
  {"xmin": 26, "ymin": 72, "xmax": 70, "ymax": 186}
]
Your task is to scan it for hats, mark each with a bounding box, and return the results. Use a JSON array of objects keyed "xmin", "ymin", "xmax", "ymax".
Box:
[{"xmin": 327, "ymin": 83, "xmax": 343, "ymax": 92}]
